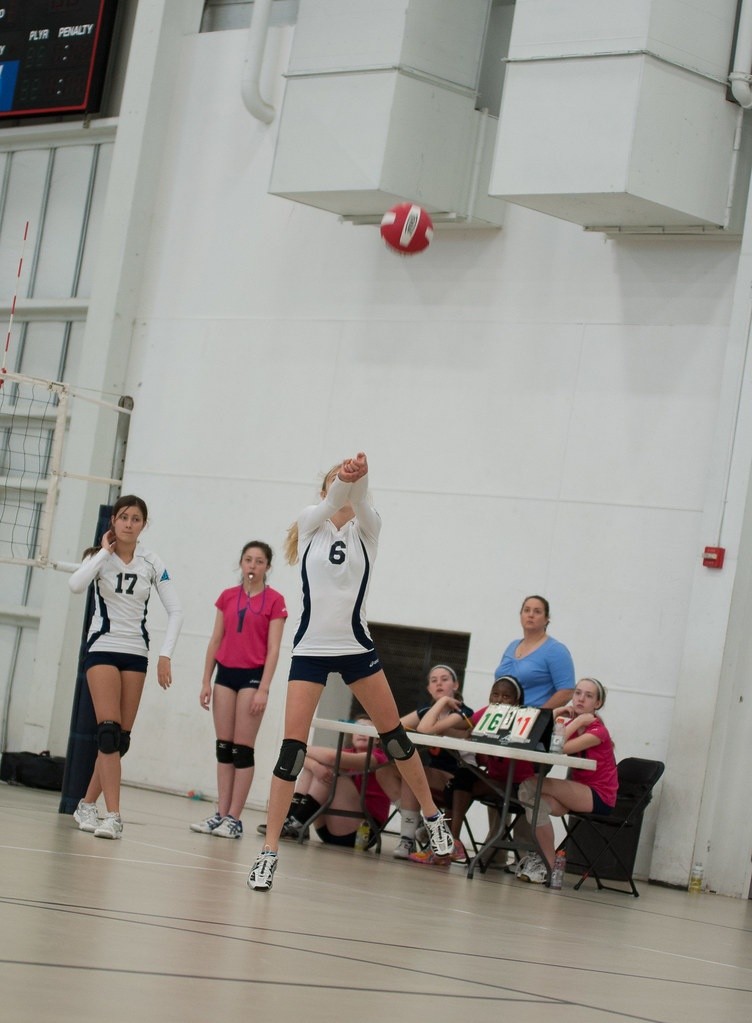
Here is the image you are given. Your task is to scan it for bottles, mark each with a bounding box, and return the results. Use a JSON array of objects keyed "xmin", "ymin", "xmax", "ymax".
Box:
[
  {"xmin": 354, "ymin": 820, "xmax": 371, "ymax": 851},
  {"xmin": 549, "ymin": 850, "xmax": 566, "ymax": 890},
  {"xmin": 688, "ymin": 862, "xmax": 704, "ymax": 895}
]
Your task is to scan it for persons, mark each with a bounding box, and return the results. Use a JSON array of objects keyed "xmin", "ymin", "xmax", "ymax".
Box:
[
  {"xmin": 257, "ymin": 715, "xmax": 391, "ymax": 851},
  {"xmin": 468, "ymin": 595, "xmax": 576, "ymax": 874},
  {"xmin": 247, "ymin": 452, "xmax": 454, "ymax": 893},
  {"xmin": 375, "ymin": 664, "xmax": 474, "ymax": 860},
  {"xmin": 68, "ymin": 495, "xmax": 183, "ymax": 840},
  {"xmin": 516, "ymin": 677, "xmax": 619, "ymax": 884},
  {"xmin": 409, "ymin": 675, "xmax": 535, "ymax": 865},
  {"xmin": 189, "ymin": 540, "xmax": 287, "ymax": 839}
]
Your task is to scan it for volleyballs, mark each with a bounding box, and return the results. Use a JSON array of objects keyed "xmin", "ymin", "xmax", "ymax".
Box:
[{"xmin": 380, "ymin": 204, "xmax": 434, "ymax": 254}]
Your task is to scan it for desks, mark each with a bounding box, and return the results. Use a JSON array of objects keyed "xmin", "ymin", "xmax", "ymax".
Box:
[{"xmin": 298, "ymin": 718, "xmax": 596, "ymax": 888}]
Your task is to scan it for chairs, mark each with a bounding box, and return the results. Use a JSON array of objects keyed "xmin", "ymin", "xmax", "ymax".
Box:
[{"xmin": 364, "ymin": 756, "xmax": 665, "ymax": 898}]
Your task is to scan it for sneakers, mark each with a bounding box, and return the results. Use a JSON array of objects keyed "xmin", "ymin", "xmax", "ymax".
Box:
[
  {"xmin": 415, "ymin": 826, "xmax": 432, "ymax": 842},
  {"xmin": 505, "ymin": 860, "xmax": 522, "ymax": 874},
  {"xmin": 190, "ymin": 813, "xmax": 227, "ymax": 834},
  {"xmin": 421, "ymin": 807, "xmax": 454, "ymax": 859},
  {"xmin": 73, "ymin": 798, "xmax": 101, "ymax": 832},
  {"xmin": 393, "ymin": 836, "xmax": 415, "ymax": 859},
  {"xmin": 409, "ymin": 836, "xmax": 467, "ymax": 866},
  {"xmin": 212, "ymin": 817, "xmax": 243, "ymax": 838},
  {"xmin": 258, "ymin": 815, "xmax": 309, "ymax": 842},
  {"xmin": 248, "ymin": 843, "xmax": 280, "ymax": 892},
  {"xmin": 95, "ymin": 812, "xmax": 123, "ymax": 838},
  {"xmin": 515, "ymin": 851, "xmax": 546, "ymax": 884}
]
